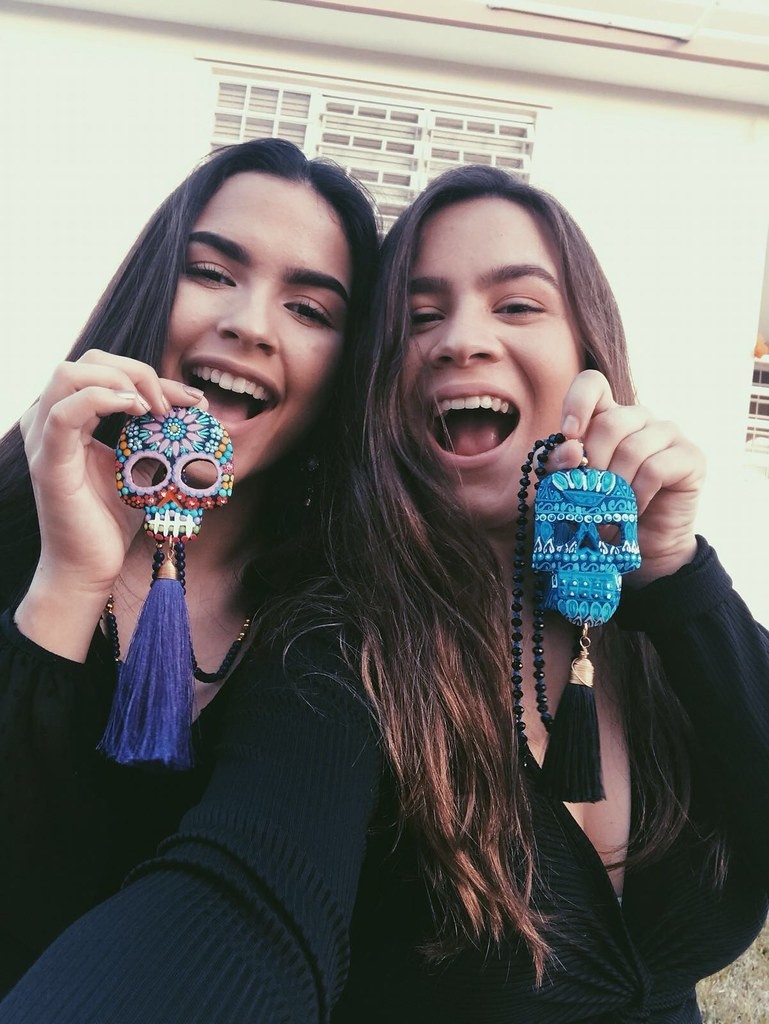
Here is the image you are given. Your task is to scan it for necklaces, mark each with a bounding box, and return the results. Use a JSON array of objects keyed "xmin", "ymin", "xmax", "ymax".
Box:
[
  {"xmin": 503, "ymin": 429, "xmax": 644, "ymax": 807},
  {"xmin": 93, "ymin": 390, "xmax": 263, "ymax": 769}
]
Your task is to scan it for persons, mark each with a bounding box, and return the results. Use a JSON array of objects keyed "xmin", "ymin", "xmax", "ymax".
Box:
[
  {"xmin": 0, "ymin": 135, "xmax": 382, "ymax": 1023},
  {"xmin": 0, "ymin": 165, "xmax": 769, "ymax": 1023}
]
